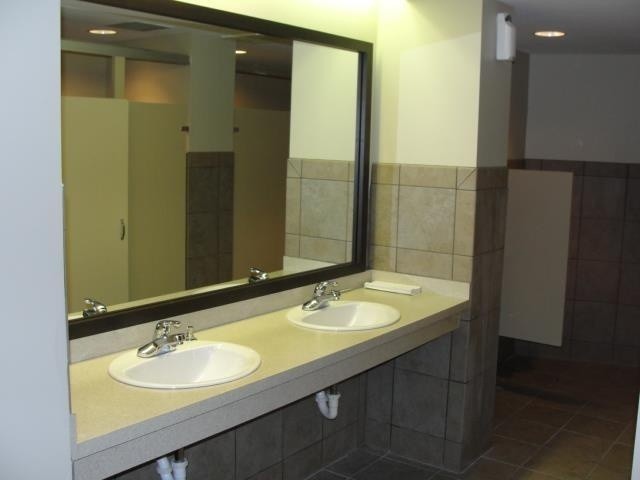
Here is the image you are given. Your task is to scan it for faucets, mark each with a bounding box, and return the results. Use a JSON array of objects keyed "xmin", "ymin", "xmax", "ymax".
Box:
[
  {"xmin": 303, "ymin": 279, "xmax": 341, "ymax": 311},
  {"xmin": 138, "ymin": 320, "xmax": 186, "ymax": 358},
  {"xmin": 83, "ymin": 298, "xmax": 108, "ymax": 316},
  {"xmin": 248, "ymin": 268, "xmax": 269, "ymax": 283}
]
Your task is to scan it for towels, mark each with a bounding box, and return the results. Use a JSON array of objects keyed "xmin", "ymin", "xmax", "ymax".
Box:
[{"xmin": 364, "ymin": 278, "xmax": 422, "ymax": 296}]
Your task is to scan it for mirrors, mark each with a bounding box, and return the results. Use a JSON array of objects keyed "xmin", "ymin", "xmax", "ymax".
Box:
[{"xmin": 61, "ymin": 0, "xmax": 373, "ymax": 336}]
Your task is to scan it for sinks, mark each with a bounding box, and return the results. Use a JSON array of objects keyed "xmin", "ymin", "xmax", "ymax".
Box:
[
  {"xmin": 109, "ymin": 340, "xmax": 261, "ymax": 390},
  {"xmin": 287, "ymin": 301, "xmax": 401, "ymax": 332},
  {"xmin": 67, "ymin": 316, "xmax": 82, "ymax": 321}
]
